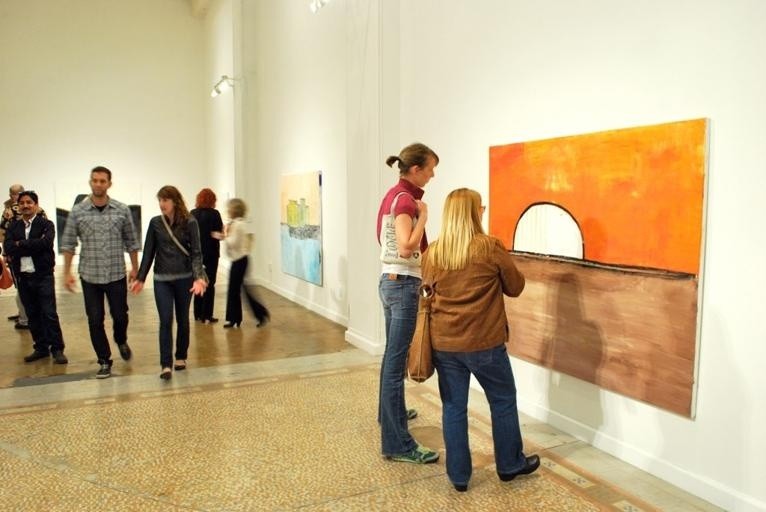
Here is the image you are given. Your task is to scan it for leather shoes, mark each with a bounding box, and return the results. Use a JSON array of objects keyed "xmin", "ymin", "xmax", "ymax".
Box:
[
  {"xmin": 496, "ymin": 453, "xmax": 541, "ymax": 482},
  {"xmin": 453, "ymin": 483, "xmax": 469, "ymax": 492},
  {"xmin": 174, "ymin": 359, "xmax": 187, "ymax": 370},
  {"xmin": 160, "ymin": 370, "xmax": 171, "ymax": 380}
]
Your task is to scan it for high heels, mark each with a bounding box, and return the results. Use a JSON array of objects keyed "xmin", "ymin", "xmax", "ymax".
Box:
[
  {"xmin": 222, "ymin": 319, "xmax": 242, "ymax": 328},
  {"xmin": 255, "ymin": 310, "xmax": 271, "ymax": 329},
  {"xmin": 194, "ymin": 314, "xmax": 219, "ymax": 324}
]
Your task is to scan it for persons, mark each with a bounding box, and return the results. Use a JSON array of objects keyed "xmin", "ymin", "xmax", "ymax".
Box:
[
  {"xmin": 130, "ymin": 185, "xmax": 209, "ymax": 382},
  {"xmin": 376, "ymin": 142, "xmax": 441, "ymax": 467},
  {"xmin": 60, "ymin": 166, "xmax": 140, "ymax": 379},
  {"xmin": 0, "ymin": 184, "xmax": 49, "ymax": 329},
  {"xmin": 420, "ymin": 187, "xmax": 541, "ymax": 492},
  {"xmin": 3, "ymin": 189, "xmax": 67, "ymax": 364},
  {"xmin": 189, "ymin": 189, "xmax": 223, "ymax": 324},
  {"xmin": 212, "ymin": 197, "xmax": 271, "ymax": 329}
]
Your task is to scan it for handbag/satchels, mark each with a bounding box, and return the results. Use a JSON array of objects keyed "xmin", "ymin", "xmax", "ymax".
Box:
[
  {"xmin": 404, "ymin": 294, "xmax": 435, "ymax": 384},
  {"xmin": 380, "ymin": 191, "xmax": 424, "ymax": 269},
  {"xmin": 0, "ymin": 255, "xmax": 16, "ymax": 290},
  {"xmin": 201, "ymin": 262, "xmax": 210, "ymax": 285}
]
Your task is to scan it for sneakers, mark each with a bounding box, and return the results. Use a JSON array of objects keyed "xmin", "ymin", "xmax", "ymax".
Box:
[
  {"xmin": 52, "ymin": 351, "xmax": 68, "ymax": 364},
  {"xmin": 22, "ymin": 350, "xmax": 50, "ymax": 363},
  {"xmin": 96, "ymin": 362, "xmax": 112, "ymax": 380},
  {"xmin": 391, "ymin": 440, "xmax": 440, "ymax": 464},
  {"xmin": 14, "ymin": 318, "xmax": 28, "ymax": 329},
  {"xmin": 404, "ymin": 408, "xmax": 418, "ymax": 420},
  {"xmin": 117, "ymin": 342, "xmax": 132, "ymax": 362}
]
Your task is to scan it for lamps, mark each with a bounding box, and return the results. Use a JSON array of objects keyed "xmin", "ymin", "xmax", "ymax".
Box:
[{"xmin": 210, "ymin": 73, "xmax": 238, "ymax": 97}]
